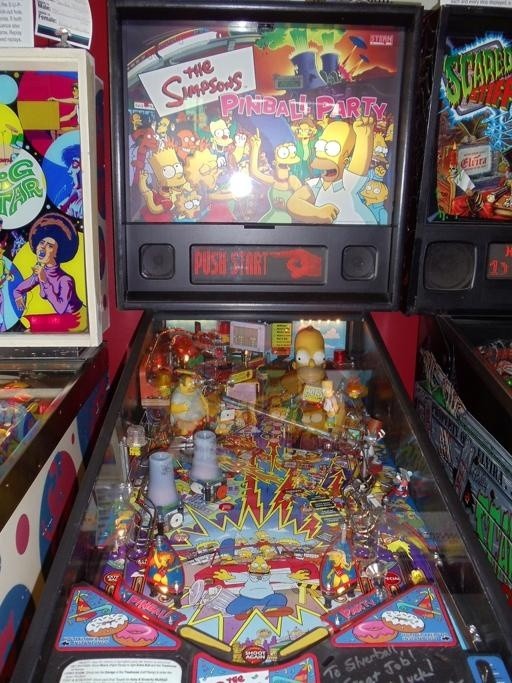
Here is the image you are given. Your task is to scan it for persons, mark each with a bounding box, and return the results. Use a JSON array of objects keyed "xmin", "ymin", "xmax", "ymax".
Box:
[
  {"xmin": 12, "ymin": 212, "xmax": 88, "ymax": 335},
  {"xmin": 169, "ymin": 375, "xmax": 209, "ymax": 435},
  {"xmin": 259, "ymin": 324, "xmax": 339, "ymax": 437}
]
